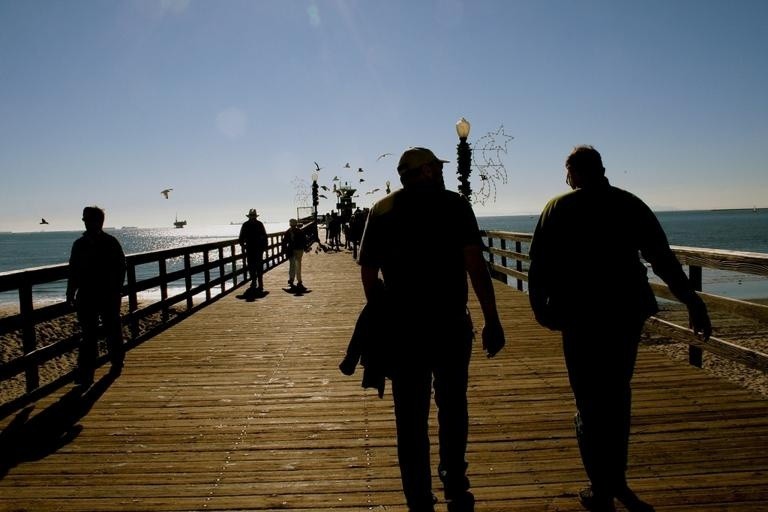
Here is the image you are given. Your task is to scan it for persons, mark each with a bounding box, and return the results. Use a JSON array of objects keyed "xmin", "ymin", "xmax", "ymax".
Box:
[
  {"xmin": 239, "ymin": 208, "xmax": 269, "ymax": 294},
  {"xmin": 280, "ymin": 218, "xmax": 310, "ymax": 288},
  {"xmin": 527, "ymin": 144, "xmax": 713, "ymax": 512},
  {"xmin": 338, "ymin": 146, "xmax": 505, "ymax": 512},
  {"xmin": 320, "ymin": 205, "xmax": 370, "ymax": 259},
  {"xmin": 64, "ymin": 208, "xmax": 126, "ymax": 384}
]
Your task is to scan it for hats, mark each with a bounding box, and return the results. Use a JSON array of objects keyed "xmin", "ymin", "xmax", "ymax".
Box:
[
  {"xmin": 245, "ymin": 209, "xmax": 259, "ymax": 217},
  {"xmin": 398, "ymin": 149, "xmax": 448, "ymax": 175}
]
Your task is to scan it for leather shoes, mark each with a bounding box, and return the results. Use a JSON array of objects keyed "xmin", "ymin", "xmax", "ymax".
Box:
[
  {"xmin": 444, "ymin": 474, "xmax": 470, "ymax": 499},
  {"xmin": 408, "ymin": 494, "xmax": 438, "ymax": 512}
]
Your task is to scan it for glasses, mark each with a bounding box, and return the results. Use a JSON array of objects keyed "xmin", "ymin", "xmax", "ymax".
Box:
[{"xmin": 566, "ymin": 174, "xmax": 571, "ymax": 185}]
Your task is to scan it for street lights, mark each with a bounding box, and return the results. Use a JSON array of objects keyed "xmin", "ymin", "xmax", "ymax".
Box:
[
  {"xmin": 454, "ymin": 115, "xmax": 475, "ymax": 207},
  {"xmin": 336, "ymin": 191, "xmax": 341, "ymax": 215},
  {"xmin": 384, "ymin": 180, "xmax": 394, "ymax": 195},
  {"xmin": 310, "ymin": 172, "xmax": 322, "ymax": 218}
]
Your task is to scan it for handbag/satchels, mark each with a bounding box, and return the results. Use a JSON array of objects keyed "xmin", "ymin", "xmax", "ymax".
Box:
[{"xmin": 286, "ymin": 246, "xmax": 293, "ymax": 259}]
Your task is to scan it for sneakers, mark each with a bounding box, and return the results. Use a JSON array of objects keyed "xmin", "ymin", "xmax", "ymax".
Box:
[
  {"xmin": 577, "ymin": 486, "xmax": 612, "ymax": 510},
  {"xmin": 110, "ymin": 365, "xmax": 122, "ymax": 375},
  {"xmin": 251, "ymin": 280, "xmax": 304, "ymax": 289}
]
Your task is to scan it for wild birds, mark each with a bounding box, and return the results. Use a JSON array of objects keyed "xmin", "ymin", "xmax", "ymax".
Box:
[
  {"xmin": 359, "ymin": 168, "xmax": 363, "ymax": 172},
  {"xmin": 344, "ymin": 163, "xmax": 350, "ymax": 168},
  {"xmin": 377, "ymin": 153, "xmax": 392, "ymax": 161},
  {"xmin": 479, "ymin": 175, "xmax": 487, "ymax": 179},
  {"xmin": 321, "ymin": 186, "xmax": 329, "ymax": 191},
  {"xmin": 314, "ymin": 162, "xmax": 322, "ymax": 171},
  {"xmin": 333, "ymin": 176, "xmax": 339, "ymax": 181},
  {"xmin": 360, "ymin": 179, "xmax": 365, "ymax": 182},
  {"xmin": 319, "ymin": 195, "xmax": 327, "ymax": 199},
  {"xmin": 40, "ymin": 219, "xmax": 48, "ymax": 224},
  {"xmin": 366, "ymin": 189, "xmax": 380, "ymax": 194},
  {"xmin": 161, "ymin": 189, "xmax": 173, "ymax": 199}
]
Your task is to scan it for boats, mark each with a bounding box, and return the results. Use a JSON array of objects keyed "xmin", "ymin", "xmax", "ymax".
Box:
[{"xmin": 172, "ymin": 212, "xmax": 188, "ymax": 228}]
